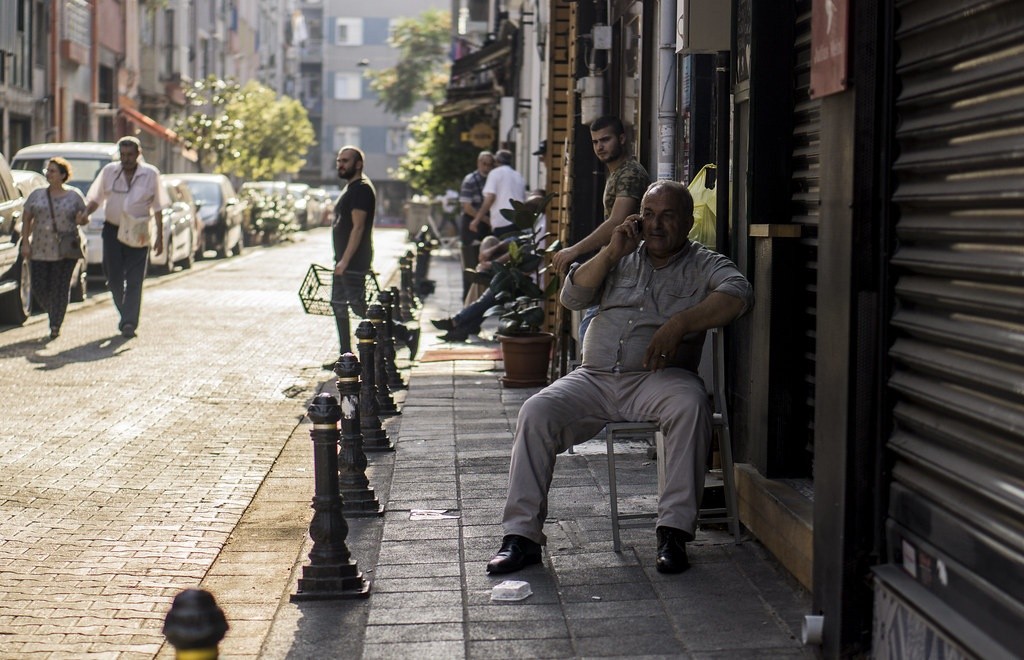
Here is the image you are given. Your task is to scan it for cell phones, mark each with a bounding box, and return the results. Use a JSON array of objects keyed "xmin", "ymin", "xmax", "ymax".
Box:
[{"xmin": 632, "ymin": 219, "xmax": 643, "ymax": 235}]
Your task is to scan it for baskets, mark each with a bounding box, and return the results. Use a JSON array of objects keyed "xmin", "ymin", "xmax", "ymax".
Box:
[{"xmin": 298, "ymin": 263, "xmax": 382, "ymax": 318}]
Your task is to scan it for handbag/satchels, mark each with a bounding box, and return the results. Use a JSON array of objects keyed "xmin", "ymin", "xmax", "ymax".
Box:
[
  {"xmin": 56, "ymin": 234, "xmax": 84, "ymax": 260},
  {"xmin": 685, "ymin": 164, "xmax": 717, "ymax": 252},
  {"xmin": 117, "ymin": 214, "xmax": 150, "ymax": 248}
]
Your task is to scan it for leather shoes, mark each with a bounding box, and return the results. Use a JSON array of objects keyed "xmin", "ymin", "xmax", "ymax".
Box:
[
  {"xmin": 656, "ymin": 533, "xmax": 687, "ymax": 572},
  {"xmin": 487, "ymin": 537, "xmax": 541, "ymax": 573}
]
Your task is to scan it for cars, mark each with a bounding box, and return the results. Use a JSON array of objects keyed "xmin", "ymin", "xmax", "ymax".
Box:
[
  {"xmin": 238, "ymin": 182, "xmax": 339, "ymax": 246},
  {"xmin": 162, "ymin": 174, "xmax": 244, "ymax": 268},
  {"xmin": 0, "ymin": 152, "xmax": 88, "ymax": 323}
]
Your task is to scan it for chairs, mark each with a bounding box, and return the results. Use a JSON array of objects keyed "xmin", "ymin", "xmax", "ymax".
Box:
[{"xmin": 605, "ymin": 323, "xmax": 743, "ymax": 556}]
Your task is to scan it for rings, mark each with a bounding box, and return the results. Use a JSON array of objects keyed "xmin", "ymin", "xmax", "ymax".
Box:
[{"xmin": 661, "ymin": 355, "xmax": 666, "ymax": 358}]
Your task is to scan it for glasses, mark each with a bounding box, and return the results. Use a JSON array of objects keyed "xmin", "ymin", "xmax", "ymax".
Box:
[{"xmin": 112, "ymin": 177, "xmax": 130, "ymax": 193}]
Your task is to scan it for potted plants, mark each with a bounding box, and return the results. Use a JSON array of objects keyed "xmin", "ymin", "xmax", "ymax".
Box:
[{"xmin": 481, "ymin": 190, "xmax": 561, "ymax": 390}]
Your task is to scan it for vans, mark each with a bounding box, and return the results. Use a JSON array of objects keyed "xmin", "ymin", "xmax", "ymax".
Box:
[{"xmin": 12, "ymin": 142, "xmax": 190, "ymax": 293}]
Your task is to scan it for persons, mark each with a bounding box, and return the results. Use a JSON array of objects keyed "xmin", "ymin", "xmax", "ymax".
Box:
[
  {"xmin": 429, "ymin": 189, "xmax": 547, "ymax": 342},
  {"xmin": 459, "ymin": 149, "xmax": 526, "ymax": 303},
  {"xmin": 321, "ymin": 147, "xmax": 421, "ymax": 370},
  {"xmin": 79, "ymin": 136, "xmax": 167, "ymax": 338},
  {"xmin": 552, "ymin": 116, "xmax": 651, "ymax": 360},
  {"xmin": 486, "ymin": 179, "xmax": 754, "ymax": 576},
  {"xmin": 22, "ymin": 157, "xmax": 89, "ymax": 339}
]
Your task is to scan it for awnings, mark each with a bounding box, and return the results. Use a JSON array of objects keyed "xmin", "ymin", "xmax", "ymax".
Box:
[
  {"xmin": 121, "ymin": 107, "xmax": 188, "ymax": 147},
  {"xmin": 433, "ymin": 37, "xmax": 515, "ymax": 118}
]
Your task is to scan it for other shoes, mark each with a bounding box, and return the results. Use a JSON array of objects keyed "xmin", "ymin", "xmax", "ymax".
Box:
[
  {"xmin": 439, "ymin": 326, "xmax": 468, "ymax": 342},
  {"xmin": 322, "ymin": 361, "xmax": 338, "ymax": 370},
  {"xmin": 406, "ymin": 328, "xmax": 420, "ymax": 360},
  {"xmin": 431, "ymin": 319, "xmax": 455, "ymax": 331},
  {"xmin": 50, "ymin": 325, "xmax": 60, "ymax": 337},
  {"xmin": 121, "ymin": 324, "xmax": 134, "ymax": 338}
]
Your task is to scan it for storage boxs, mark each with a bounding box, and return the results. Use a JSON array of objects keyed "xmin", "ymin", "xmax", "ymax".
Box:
[{"xmin": 299, "ymin": 263, "xmax": 381, "ymax": 319}]
